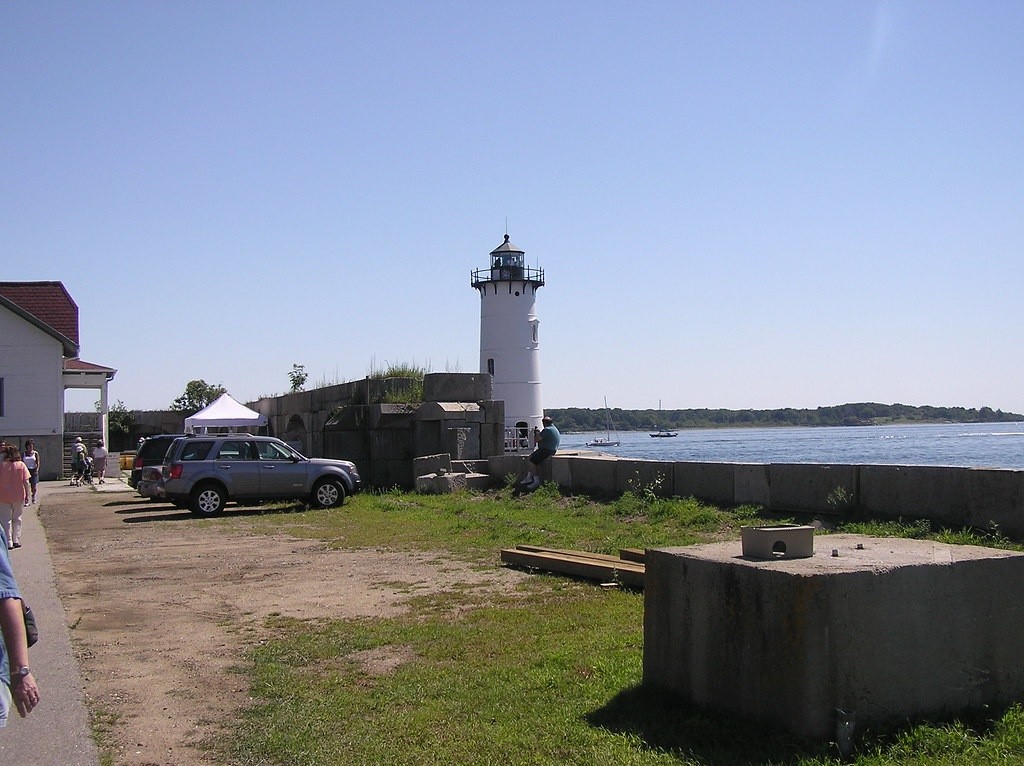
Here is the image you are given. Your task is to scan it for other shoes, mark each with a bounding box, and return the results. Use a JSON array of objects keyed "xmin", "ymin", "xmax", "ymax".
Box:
[
  {"xmin": 70, "ymin": 482, "xmax": 75, "ymax": 485},
  {"xmin": 23, "ymin": 502, "xmax": 31, "ymax": 507},
  {"xmin": 101, "ymin": 479, "xmax": 106, "ymax": 483},
  {"xmin": 99, "ymin": 481, "xmax": 102, "ymax": 484},
  {"xmin": 77, "ymin": 480, "xmax": 80, "ymax": 487},
  {"xmin": 527, "ymin": 481, "xmax": 540, "ymax": 488},
  {"xmin": 521, "ymin": 479, "xmax": 533, "ymax": 484},
  {"xmin": 13, "ymin": 541, "xmax": 21, "ymax": 547},
  {"xmin": 32, "ymin": 495, "xmax": 36, "ymax": 504},
  {"xmin": 8, "ymin": 545, "xmax": 13, "ymax": 549}
]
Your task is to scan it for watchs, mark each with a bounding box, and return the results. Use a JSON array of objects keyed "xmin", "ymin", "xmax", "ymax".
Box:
[{"xmin": 11, "ymin": 666, "xmax": 29, "ymax": 677}]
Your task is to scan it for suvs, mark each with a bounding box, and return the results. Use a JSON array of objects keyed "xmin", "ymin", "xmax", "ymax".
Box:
[
  {"xmin": 128, "ymin": 434, "xmax": 187, "ymax": 489},
  {"xmin": 158, "ymin": 432, "xmax": 363, "ymax": 517}
]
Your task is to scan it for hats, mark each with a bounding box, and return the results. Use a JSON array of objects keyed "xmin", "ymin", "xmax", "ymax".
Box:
[
  {"xmin": 76, "ymin": 446, "xmax": 84, "ymax": 453},
  {"xmin": 76, "ymin": 437, "xmax": 82, "ymax": 442}
]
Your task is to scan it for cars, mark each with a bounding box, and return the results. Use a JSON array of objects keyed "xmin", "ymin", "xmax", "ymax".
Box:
[{"xmin": 136, "ymin": 464, "xmax": 176, "ymax": 501}]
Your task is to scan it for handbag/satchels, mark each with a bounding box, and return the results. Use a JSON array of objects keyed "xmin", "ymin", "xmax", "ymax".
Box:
[{"xmin": 21, "ymin": 599, "xmax": 38, "ymax": 648}]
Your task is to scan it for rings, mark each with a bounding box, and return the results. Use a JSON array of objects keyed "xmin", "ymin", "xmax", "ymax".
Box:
[{"xmin": 31, "ymin": 696, "xmax": 37, "ymax": 701}]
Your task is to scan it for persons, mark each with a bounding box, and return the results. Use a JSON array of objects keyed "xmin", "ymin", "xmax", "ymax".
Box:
[
  {"xmin": 0, "ymin": 525, "xmax": 42, "ymax": 729},
  {"xmin": 70, "ymin": 436, "xmax": 109, "ymax": 487},
  {"xmin": 0, "ymin": 441, "xmax": 31, "ymax": 549},
  {"xmin": 22, "ymin": 439, "xmax": 40, "ymax": 506},
  {"xmin": 519, "ymin": 416, "xmax": 559, "ymax": 490}
]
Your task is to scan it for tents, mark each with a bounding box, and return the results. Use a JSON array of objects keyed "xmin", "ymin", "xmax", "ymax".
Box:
[{"xmin": 184, "ymin": 393, "xmax": 269, "ymax": 437}]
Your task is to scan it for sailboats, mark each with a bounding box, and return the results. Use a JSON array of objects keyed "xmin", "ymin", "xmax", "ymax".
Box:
[
  {"xmin": 648, "ymin": 399, "xmax": 679, "ymax": 438},
  {"xmin": 585, "ymin": 397, "xmax": 622, "ymax": 446}
]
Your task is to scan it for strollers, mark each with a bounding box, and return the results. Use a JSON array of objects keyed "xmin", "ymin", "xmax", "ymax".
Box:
[{"xmin": 76, "ymin": 458, "xmax": 94, "ymax": 483}]
